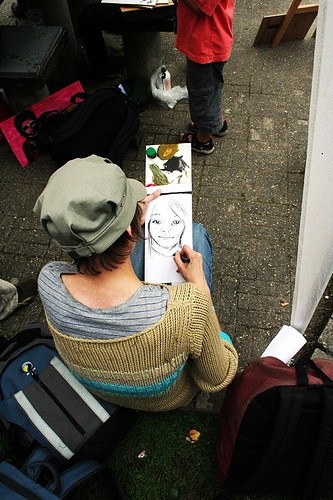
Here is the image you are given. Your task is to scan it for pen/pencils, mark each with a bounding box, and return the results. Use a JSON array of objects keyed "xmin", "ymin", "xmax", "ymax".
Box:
[{"xmin": 173, "ymin": 254, "xmax": 190, "ymax": 263}]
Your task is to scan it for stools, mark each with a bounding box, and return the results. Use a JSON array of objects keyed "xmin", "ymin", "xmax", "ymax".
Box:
[{"xmin": 123, "ymin": 30, "xmax": 162, "ymax": 104}]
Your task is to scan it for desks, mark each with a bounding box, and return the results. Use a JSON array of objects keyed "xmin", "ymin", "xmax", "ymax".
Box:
[{"xmin": 0, "ymin": 24, "xmax": 66, "ymax": 110}]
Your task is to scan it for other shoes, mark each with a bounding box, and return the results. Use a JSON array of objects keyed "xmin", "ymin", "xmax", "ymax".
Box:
[{"xmin": 130, "ymin": 95, "xmax": 153, "ymax": 113}]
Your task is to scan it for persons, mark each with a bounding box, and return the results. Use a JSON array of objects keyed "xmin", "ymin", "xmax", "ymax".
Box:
[
  {"xmin": 174, "ymin": 0, "xmax": 235, "ymax": 154},
  {"xmin": 33, "ymin": 154, "xmax": 239, "ymax": 412}
]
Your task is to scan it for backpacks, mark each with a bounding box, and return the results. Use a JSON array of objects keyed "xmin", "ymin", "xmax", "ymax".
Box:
[
  {"xmin": 38, "ymin": 88, "xmax": 140, "ymax": 170},
  {"xmin": 0, "ymin": 326, "xmax": 117, "ymax": 500}
]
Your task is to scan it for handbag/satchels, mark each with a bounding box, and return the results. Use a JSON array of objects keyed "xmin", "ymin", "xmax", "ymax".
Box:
[{"xmin": 219, "ymin": 356, "xmax": 333, "ymax": 500}]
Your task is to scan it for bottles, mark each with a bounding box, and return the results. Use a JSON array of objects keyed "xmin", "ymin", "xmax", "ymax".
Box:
[{"xmin": 157, "ymin": 68, "xmax": 171, "ymax": 91}]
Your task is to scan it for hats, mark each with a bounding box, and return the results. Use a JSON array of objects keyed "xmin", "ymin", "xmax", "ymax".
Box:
[{"xmin": 32, "ymin": 154, "xmax": 148, "ymax": 258}]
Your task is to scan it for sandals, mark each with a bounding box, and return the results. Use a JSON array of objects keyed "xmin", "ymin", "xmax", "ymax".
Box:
[
  {"xmin": 188, "ymin": 115, "xmax": 228, "ymax": 137},
  {"xmin": 177, "ymin": 131, "xmax": 215, "ymax": 154}
]
